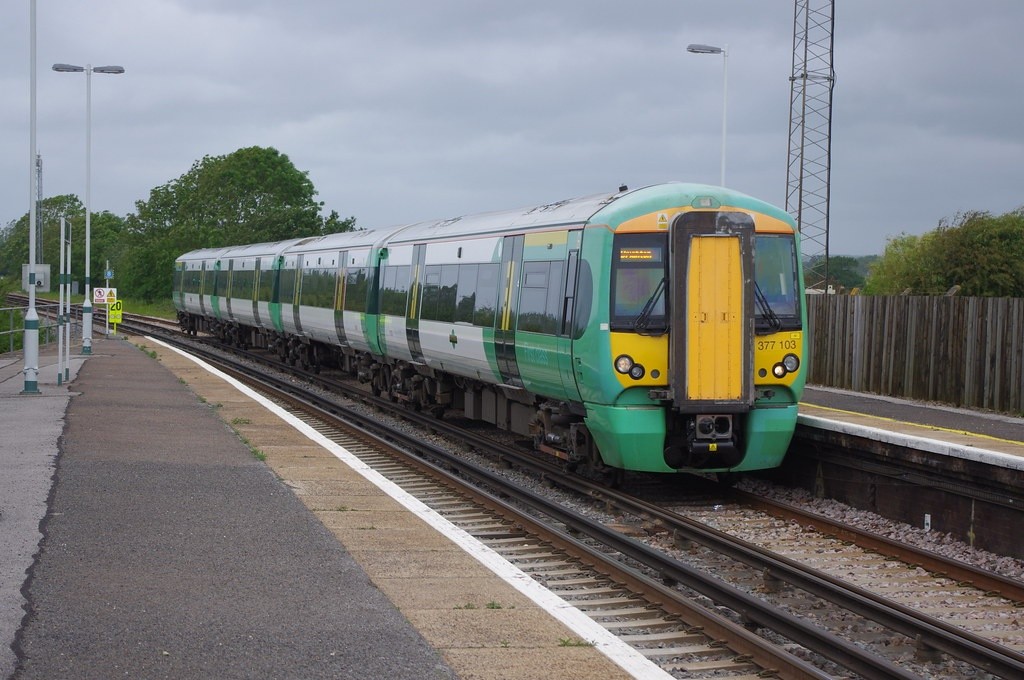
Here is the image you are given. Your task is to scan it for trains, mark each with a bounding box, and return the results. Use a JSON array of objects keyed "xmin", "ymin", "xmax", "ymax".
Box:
[{"xmin": 172, "ymin": 180, "xmax": 809, "ymax": 493}]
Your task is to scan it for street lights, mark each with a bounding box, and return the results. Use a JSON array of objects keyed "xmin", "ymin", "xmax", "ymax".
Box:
[
  {"xmin": 52, "ymin": 62, "xmax": 125, "ymax": 353},
  {"xmin": 687, "ymin": 43, "xmax": 729, "ymax": 188}
]
